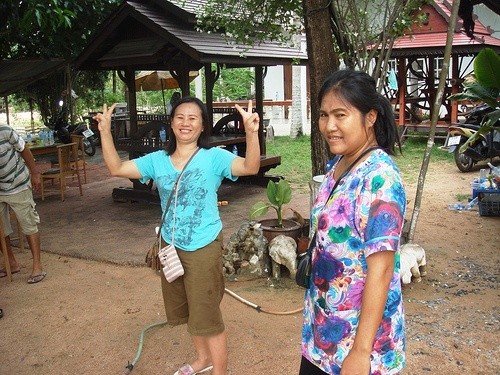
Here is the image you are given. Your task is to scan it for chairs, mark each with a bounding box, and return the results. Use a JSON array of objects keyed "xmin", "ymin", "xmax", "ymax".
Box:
[{"xmin": 0, "ymin": 134, "xmax": 85, "ymax": 283}]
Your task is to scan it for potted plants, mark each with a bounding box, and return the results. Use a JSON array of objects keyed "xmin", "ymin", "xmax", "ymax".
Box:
[
  {"xmin": 249, "ymin": 178, "xmax": 302, "ymax": 252},
  {"xmin": 289, "ymin": 208, "xmax": 310, "ymax": 255}
]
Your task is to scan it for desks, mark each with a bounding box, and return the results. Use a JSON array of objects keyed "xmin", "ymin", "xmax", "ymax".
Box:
[{"xmin": 24, "ymin": 142, "xmax": 64, "ymax": 155}]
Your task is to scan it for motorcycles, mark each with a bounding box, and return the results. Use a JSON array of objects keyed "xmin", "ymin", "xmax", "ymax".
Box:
[
  {"xmin": 46, "ymin": 100, "xmax": 96, "ymax": 156},
  {"xmin": 440, "ymin": 101, "xmax": 500, "ymax": 173}
]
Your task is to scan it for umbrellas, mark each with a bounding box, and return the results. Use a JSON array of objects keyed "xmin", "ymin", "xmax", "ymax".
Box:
[{"xmin": 135, "ymin": 70, "xmax": 199, "ymax": 115}]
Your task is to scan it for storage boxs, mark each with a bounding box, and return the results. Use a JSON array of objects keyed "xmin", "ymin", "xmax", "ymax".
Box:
[{"xmin": 479, "ymin": 190, "xmax": 500, "ymax": 216}]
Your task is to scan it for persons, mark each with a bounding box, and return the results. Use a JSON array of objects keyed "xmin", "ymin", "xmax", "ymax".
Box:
[
  {"xmin": 93, "ymin": 96, "xmax": 260, "ymax": 375},
  {"xmin": 0, "ymin": 123, "xmax": 46, "ymax": 283},
  {"xmin": 299, "ymin": 68, "xmax": 406, "ymax": 375},
  {"xmin": 166, "ymin": 92, "xmax": 180, "ymax": 115}
]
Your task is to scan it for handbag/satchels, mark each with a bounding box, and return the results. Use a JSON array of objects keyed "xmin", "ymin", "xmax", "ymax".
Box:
[
  {"xmin": 145, "ymin": 240, "xmax": 160, "ymax": 271},
  {"xmin": 157, "ymin": 244, "xmax": 185, "ymax": 284},
  {"xmin": 295, "ymin": 250, "xmax": 313, "ymax": 288}
]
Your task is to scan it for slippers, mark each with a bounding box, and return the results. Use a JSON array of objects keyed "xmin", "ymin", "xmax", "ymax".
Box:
[
  {"xmin": 27, "ymin": 271, "xmax": 47, "ymax": 283},
  {"xmin": 173, "ymin": 363, "xmax": 213, "ymax": 375},
  {"xmin": 0, "ymin": 266, "xmax": 21, "ymax": 278}
]
